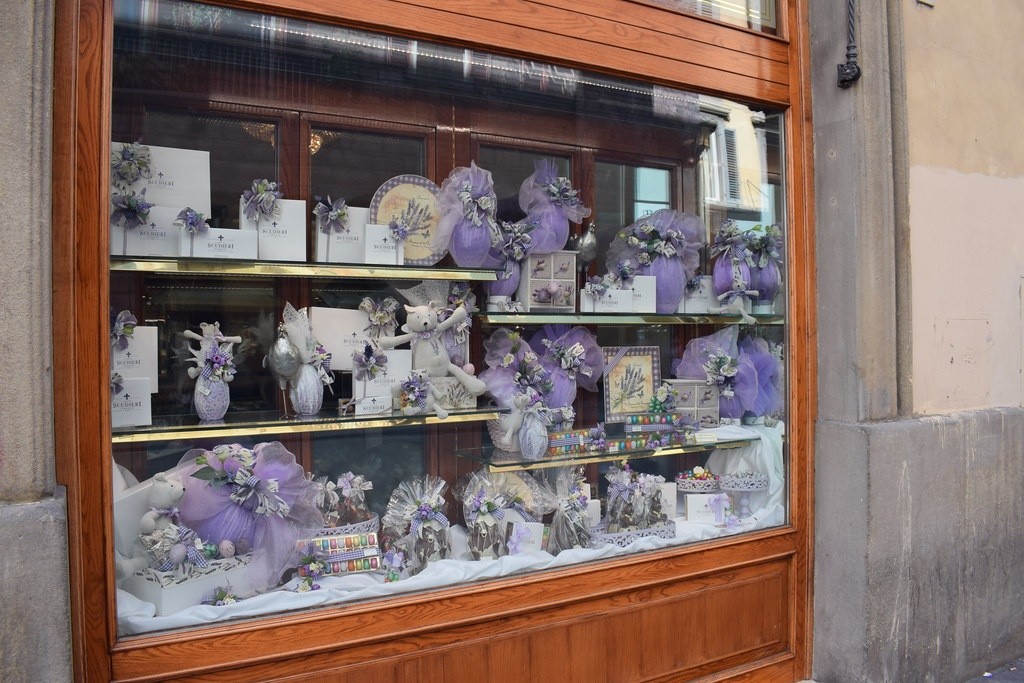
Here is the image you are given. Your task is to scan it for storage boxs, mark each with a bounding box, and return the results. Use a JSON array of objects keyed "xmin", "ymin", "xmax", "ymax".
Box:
[
  {"xmin": 110, "ymin": 140, "xmax": 211, "ymax": 222},
  {"xmin": 658, "ymin": 480, "xmax": 677, "ymax": 521},
  {"xmin": 514, "ymin": 248, "xmax": 581, "ymax": 313},
  {"xmin": 114, "ymin": 452, "xmax": 270, "ymax": 619},
  {"xmin": 576, "ymin": 287, "xmax": 635, "ymax": 313},
  {"xmin": 365, "ymin": 223, "xmax": 406, "ymax": 266},
  {"xmin": 240, "ymin": 195, "xmax": 307, "ymax": 264},
  {"xmin": 109, "ymin": 326, "xmax": 159, "ymax": 395},
  {"xmin": 186, "ymin": 227, "xmax": 259, "ymax": 259},
  {"xmin": 355, "ymin": 396, "xmax": 393, "ymax": 419},
  {"xmin": 662, "ymin": 378, "xmax": 720, "ymax": 430},
  {"xmin": 112, "ymin": 395, "xmax": 152, "ymax": 428},
  {"xmin": 674, "ymin": 275, "xmax": 721, "ymax": 316},
  {"xmin": 312, "ymin": 198, "xmax": 372, "ymax": 263},
  {"xmin": 353, "ymin": 349, "xmax": 412, "ymax": 399},
  {"xmin": 108, "ymin": 205, "xmax": 189, "ymax": 258},
  {"xmin": 603, "ymin": 274, "xmax": 657, "ymax": 313},
  {"xmin": 308, "ymin": 307, "xmax": 395, "ymax": 371},
  {"xmin": 683, "ymin": 492, "xmax": 728, "ymax": 527}
]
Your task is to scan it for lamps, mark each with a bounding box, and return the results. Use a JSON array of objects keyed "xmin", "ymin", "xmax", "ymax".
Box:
[{"xmin": 242, "ymin": 120, "xmax": 343, "ymax": 157}]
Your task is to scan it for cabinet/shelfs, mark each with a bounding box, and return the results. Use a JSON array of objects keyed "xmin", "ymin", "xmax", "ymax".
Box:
[{"xmin": 54, "ymin": 0, "xmax": 813, "ymax": 683}]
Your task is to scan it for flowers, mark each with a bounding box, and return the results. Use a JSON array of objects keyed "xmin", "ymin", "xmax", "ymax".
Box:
[
  {"xmin": 109, "ymin": 143, "xmax": 785, "ymax": 606},
  {"xmin": 503, "ymin": 327, "xmax": 576, "ymax": 428}
]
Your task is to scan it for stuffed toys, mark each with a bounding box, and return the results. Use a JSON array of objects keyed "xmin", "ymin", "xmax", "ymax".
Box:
[
  {"xmin": 500, "ymin": 392, "xmax": 542, "ymax": 446},
  {"xmin": 184, "ymin": 322, "xmax": 242, "ymax": 382},
  {"xmin": 378, "ymin": 300, "xmax": 486, "ymax": 396},
  {"xmin": 116, "ymin": 472, "xmax": 209, "ymax": 581},
  {"xmin": 404, "ymin": 369, "xmax": 448, "ymax": 419},
  {"xmin": 707, "ymin": 279, "xmax": 759, "ymax": 324}
]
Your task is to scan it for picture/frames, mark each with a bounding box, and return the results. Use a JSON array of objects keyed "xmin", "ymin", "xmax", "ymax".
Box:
[{"xmin": 601, "ymin": 345, "xmax": 662, "ymax": 425}]
